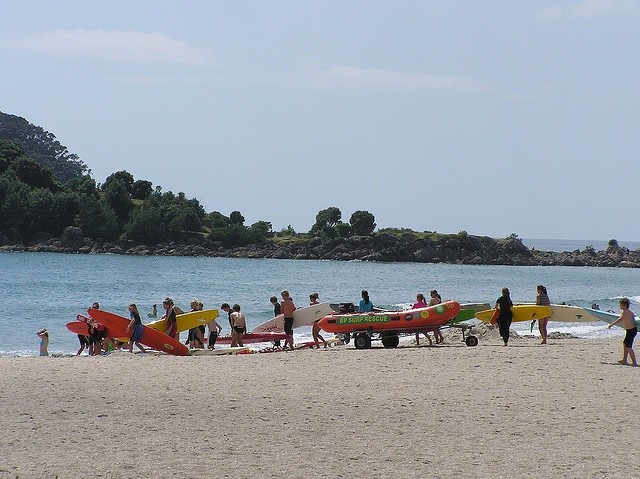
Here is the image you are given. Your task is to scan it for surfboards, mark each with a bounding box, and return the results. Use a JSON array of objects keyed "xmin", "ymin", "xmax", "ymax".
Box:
[
  {"xmin": 548, "ymin": 304, "xmax": 601, "ymax": 322},
  {"xmin": 203, "ymin": 332, "xmax": 292, "ymax": 344},
  {"xmin": 68, "ymin": 322, "xmax": 126, "ymax": 339},
  {"xmin": 116, "ymin": 309, "xmax": 219, "ymax": 342},
  {"xmin": 88, "ymin": 308, "xmax": 189, "ymax": 355},
  {"xmin": 447, "ymin": 303, "xmax": 491, "ymax": 324},
  {"xmin": 582, "ymin": 306, "xmax": 640, "ymax": 331},
  {"xmin": 189, "ymin": 347, "xmax": 250, "ymax": 355},
  {"xmin": 474, "ymin": 305, "xmax": 554, "ymax": 322},
  {"xmin": 78, "ymin": 315, "xmax": 104, "ymax": 331},
  {"xmin": 329, "ymin": 303, "xmax": 388, "ymax": 314},
  {"xmin": 251, "ymin": 301, "xmax": 334, "ymax": 334},
  {"xmin": 256, "ymin": 338, "xmax": 339, "ymax": 353}
]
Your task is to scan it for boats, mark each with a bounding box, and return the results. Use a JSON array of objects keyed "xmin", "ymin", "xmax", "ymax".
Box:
[{"xmin": 317, "ymin": 300, "xmax": 478, "ymax": 349}]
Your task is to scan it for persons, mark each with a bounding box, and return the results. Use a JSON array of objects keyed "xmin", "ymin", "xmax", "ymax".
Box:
[
  {"xmin": 608, "ymin": 298, "xmax": 638, "ymax": 366},
  {"xmin": 162, "ymin": 301, "xmax": 177, "ymax": 339},
  {"xmin": 412, "ymin": 293, "xmax": 432, "ymax": 346},
  {"xmin": 430, "ymin": 290, "xmax": 444, "ymax": 344},
  {"xmin": 310, "ymin": 293, "xmax": 326, "ymax": 348},
  {"xmin": 127, "ymin": 304, "xmax": 145, "ymax": 354},
  {"xmin": 37, "ymin": 329, "xmax": 48, "ymax": 357},
  {"xmin": 189, "ymin": 301, "xmax": 207, "ymax": 350},
  {"xmin": 165, "ymin": 297, "xmax": 184, "ymax": 341},
  {"xmin": 75, "ymin": 301, "xmax": 129, "ymax": 356},
  {"xmin": 536, "ymin": 285, "xmax": 553, "ymax": 344},
  {"xmin": 208, "ymin": 319, "xmax": 222, "ymax": 349},
  {"xmin": 221, "ymin": 303, "xmax": 234, "ymax": 333},
  {"xmin": 281, "ymin": 291, "xmax": 296, "ymax": 348},
  {"xmin": 270, "ymin": 296, "xmax": 282, "ymax": 347},
  {"xmin": 496, "ymin": 287, "xmax": 513, "ymax": 345},
  {"xmin": 230, "ymin": 304, "xmax": 247, "ymax": 346},
  {"xmin": 151, "ymin": 304, "xmax": 157, "ymax": 316},
  {"xmin": 357, "ymin": 290, "xmax": 374, "ymax": 313}
]
